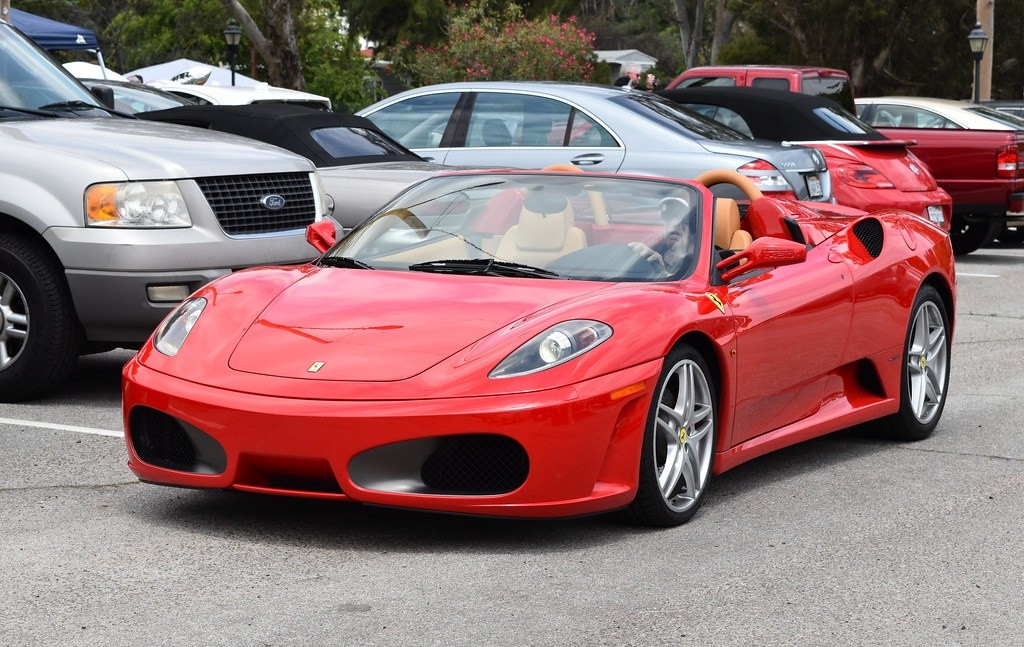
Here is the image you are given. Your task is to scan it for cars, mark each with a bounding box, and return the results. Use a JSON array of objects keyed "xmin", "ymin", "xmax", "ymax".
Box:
[
  {"xmin": 66, "ymin": 80, "xmax": 953, "ymax": 233},
  {"xmin": 849, "ymin": 96, "xmax": 1024, "ymax": 255}
]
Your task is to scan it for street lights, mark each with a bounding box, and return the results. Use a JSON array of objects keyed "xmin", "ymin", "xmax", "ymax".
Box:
[
  {"xmin": 223, "ymin": 20, "xmax": 244, "ymax": 86},
  {"xmin": 965, "ymin": 18, "xmax": 989, "ymax": 101}
]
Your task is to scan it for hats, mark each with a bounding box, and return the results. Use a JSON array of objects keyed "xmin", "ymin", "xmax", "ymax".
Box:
[{"xmin": 657, "ymin": 187, "xmax": 691, "ymax": 208}]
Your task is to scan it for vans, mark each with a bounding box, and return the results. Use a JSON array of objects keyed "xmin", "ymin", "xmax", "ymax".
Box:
[{"xmin": 663, "ymin": 63, "xmax": 858, "ymax": 122}]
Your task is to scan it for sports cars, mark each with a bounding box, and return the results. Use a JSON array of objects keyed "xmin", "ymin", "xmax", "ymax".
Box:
[{"xmin": 121, "ymin": 171, "xmax": 958, "ymax": 530}]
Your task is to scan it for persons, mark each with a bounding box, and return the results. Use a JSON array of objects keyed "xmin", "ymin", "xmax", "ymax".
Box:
[
  {"xmin": 614, "ymin": 65, "xmax": 655, "ymax": 92},
  {"xmin": 626, "ymin": 184, "xmax": 740, "ymax": 287}
]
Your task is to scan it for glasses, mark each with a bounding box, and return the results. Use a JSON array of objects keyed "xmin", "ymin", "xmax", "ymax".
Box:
[{"xmin": 660, "ymin": 210, "xmax": 692, "ymax": 224}]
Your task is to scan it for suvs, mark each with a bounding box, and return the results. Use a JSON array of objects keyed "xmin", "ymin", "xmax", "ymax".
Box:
[{"xmin": 0, "ymin": 18, "xmax": 347, "ymax": 403}]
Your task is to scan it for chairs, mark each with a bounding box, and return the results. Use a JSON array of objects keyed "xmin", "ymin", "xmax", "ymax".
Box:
[
  {"xmin": 495, "ymin": 197, "xmax": 587, "ymax": 268},
  {"xmin": 704, "ymin": 110, "xmax": 725, "ymax": 125},
  {"xmin": 876, "ymin": 111, "xmax": 898, "ymax": 126},
  {"xmin": 482, "ymin": 118, "xmax": 512, "ymax": 145},
  {"xmin": 715, "ymin": 198, "xmax": 753, "ymax": 265}
]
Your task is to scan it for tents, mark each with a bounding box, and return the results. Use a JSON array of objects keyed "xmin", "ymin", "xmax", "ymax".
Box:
[{"xmin": 6, "ymin": 5, "xmax": 109, "ymax": 81}]
